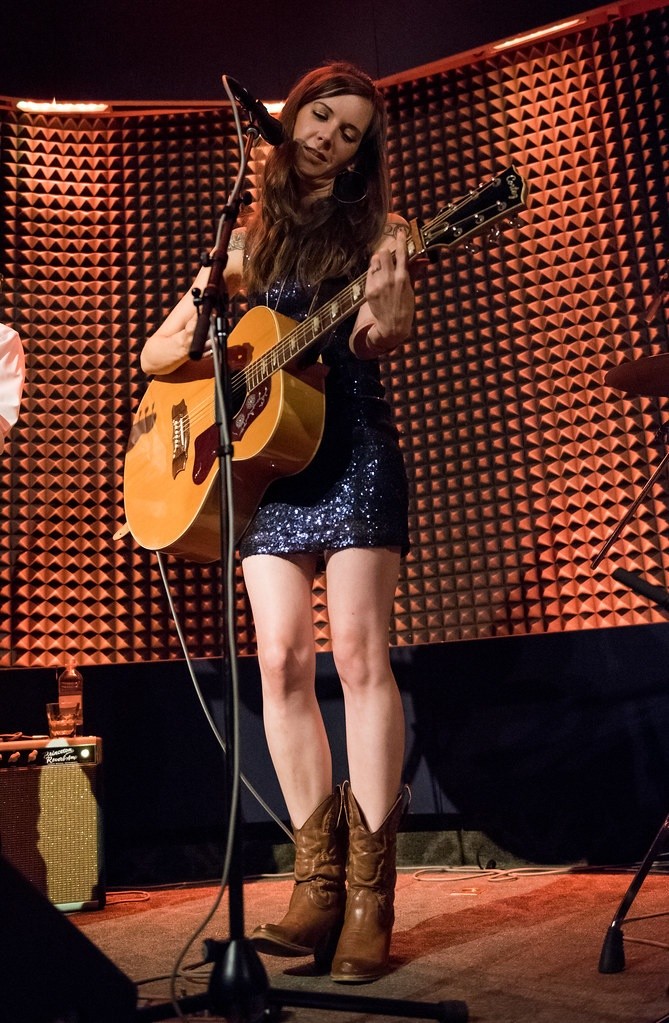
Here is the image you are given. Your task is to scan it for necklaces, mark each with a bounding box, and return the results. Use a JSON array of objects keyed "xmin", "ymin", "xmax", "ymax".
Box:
[{"xmin": 266, "ymin": 237, "xmax": 304, "ymax": 312}]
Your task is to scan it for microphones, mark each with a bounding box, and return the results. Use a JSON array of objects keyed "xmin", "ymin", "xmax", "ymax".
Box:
[{"xmin": 221, "ymin": 77, "xmax": 286, "ymax": 145}]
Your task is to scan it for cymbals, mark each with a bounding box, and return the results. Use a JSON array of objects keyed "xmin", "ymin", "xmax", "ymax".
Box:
[{"xmin": 604, "ymin": 351, "xmax": 669, "ymax": 397}]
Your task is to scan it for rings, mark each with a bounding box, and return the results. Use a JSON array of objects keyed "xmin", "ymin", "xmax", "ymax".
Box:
[{"xmin": 371, "ymin": 267, "xmax": 381, "ymax": 275}]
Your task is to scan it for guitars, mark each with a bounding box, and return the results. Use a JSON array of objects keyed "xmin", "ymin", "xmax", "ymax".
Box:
[{"xmin": 121, "ymin": 161, "xmax": 532, "ymax": 568}]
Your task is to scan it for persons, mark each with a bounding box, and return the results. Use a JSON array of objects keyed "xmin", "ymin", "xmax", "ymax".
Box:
[{"xmin": 141, "ymin": 64, "xmax": 416, "ymax": 980}]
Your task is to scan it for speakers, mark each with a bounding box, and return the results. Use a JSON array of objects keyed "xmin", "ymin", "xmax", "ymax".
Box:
[
  {"xmin": 0, "ymin": 734, "xmax": 106, "ymax": 913},
  {"xmin": 0, "ymin": 853, "xmax": 138, "ymax": 1023}
]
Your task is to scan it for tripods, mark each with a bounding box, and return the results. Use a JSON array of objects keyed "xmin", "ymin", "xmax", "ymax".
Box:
[{"xmin": 130, "ymin": 109, "xmax": 466, "ymax": 1023}]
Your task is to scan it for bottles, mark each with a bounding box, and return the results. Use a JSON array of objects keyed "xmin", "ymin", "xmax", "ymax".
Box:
[{"xmin": 58, "ymin": 657, "xmax": 83, "ymax": 736}]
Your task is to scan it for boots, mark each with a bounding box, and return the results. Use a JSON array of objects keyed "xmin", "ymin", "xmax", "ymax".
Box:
[
  {"xmin": 328, "ymin": 784, "xmax": 413, "ymax": 982},
  {"xmin": 248, "ymin": 784, "xmax": 348, "ymax": 966}
]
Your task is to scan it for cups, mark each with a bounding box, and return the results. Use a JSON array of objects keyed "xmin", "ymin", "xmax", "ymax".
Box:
[{"xmin": 46, "ymin": 702, "xmax": 78, "ymax": 739}]
